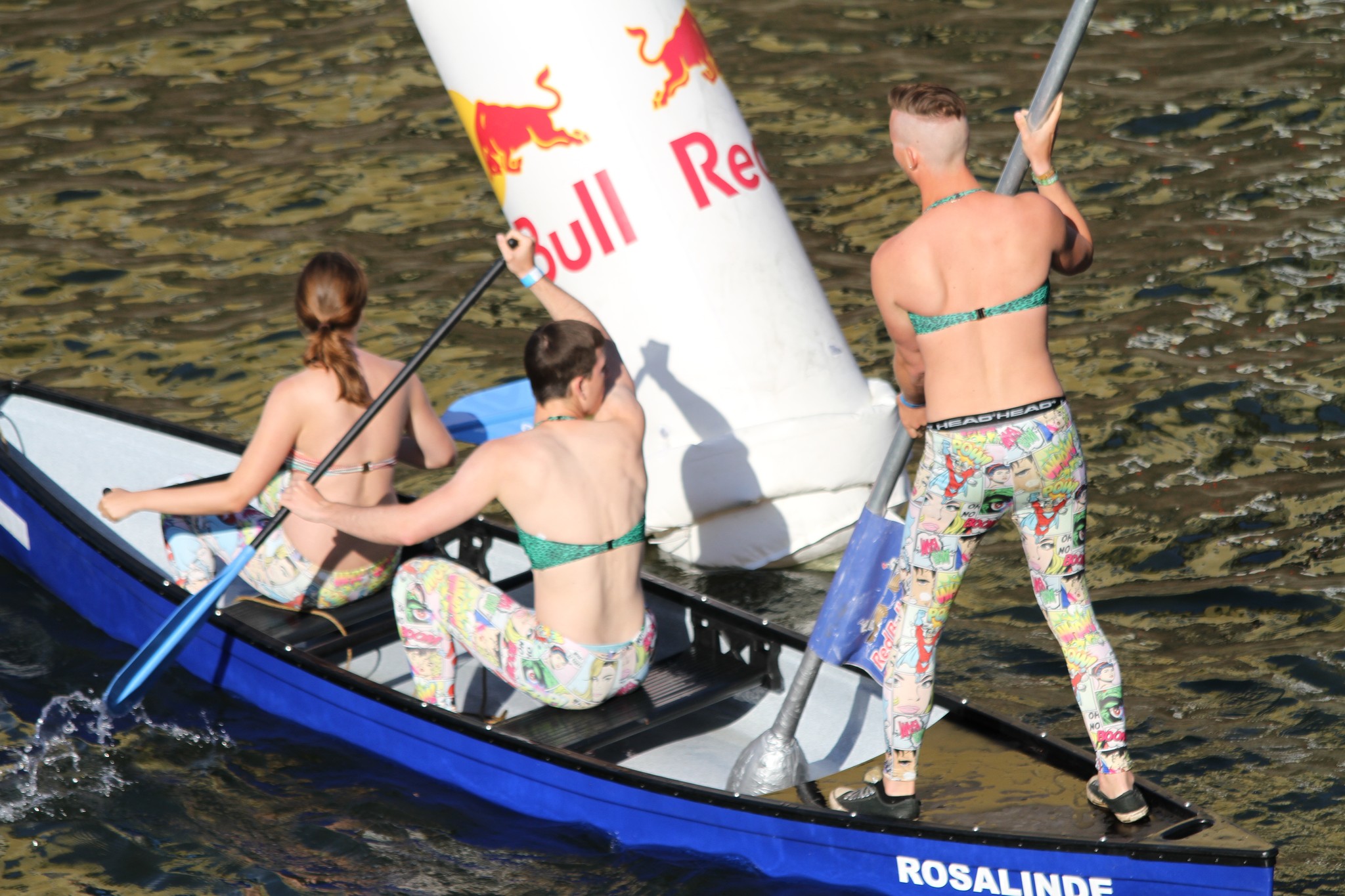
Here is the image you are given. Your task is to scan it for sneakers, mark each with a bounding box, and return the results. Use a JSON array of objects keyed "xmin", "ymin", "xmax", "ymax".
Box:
[
  {"xmin": 1086, "ymin": 774, "xmax": 1148, "ymax": 823},
  {"xmin": 826, "ymin": 783, "xmax": 922, "ymax": 822}
]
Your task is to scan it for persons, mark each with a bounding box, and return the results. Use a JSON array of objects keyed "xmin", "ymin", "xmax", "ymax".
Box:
[
  {"xmin": 98, "ymin": 246, "xmax": 454, "ymax": 616},
  {"xmin": 826, "ymin": 82, "xmax": 1148, "ymax": 828},
  {"xmin": 280, "ymin": 226, "xmax": 658, "ymax": 712}
]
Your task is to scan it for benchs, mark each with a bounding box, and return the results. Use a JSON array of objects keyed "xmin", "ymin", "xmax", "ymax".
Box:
[
  {"xmin": 485, "ymin": 646, "xmax": 768, "ymax": 759},
  {"xmin": 218, "ymin": 540, "xmax": 454, "ymax": 663}
]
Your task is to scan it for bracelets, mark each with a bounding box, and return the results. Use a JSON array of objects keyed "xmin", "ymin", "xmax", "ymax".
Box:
[
  {"xmin": 1030, "ymin": 165, "xmax": 1059, "ymax": 185},
  {"xmin": 899, "ymin": 393, "xmax": 925, "ymax": 409},
  {"xmin": 521, "ymin": 267, "xmax": 546, "ymax": 287}
]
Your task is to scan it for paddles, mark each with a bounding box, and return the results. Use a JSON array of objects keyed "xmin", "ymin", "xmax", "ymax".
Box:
[
  {"xmin": 103, "ymin": 238, "xmax": 519, "ymax": 720},
  {"xmin": 105, "ymin": 377, "xmax": 538, "ymax": 507}
]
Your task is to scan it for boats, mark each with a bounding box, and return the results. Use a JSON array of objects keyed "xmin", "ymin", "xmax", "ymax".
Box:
[{"xmin": 0, "ymin": 377, "xmax": 1279, "ymax": 896}]
